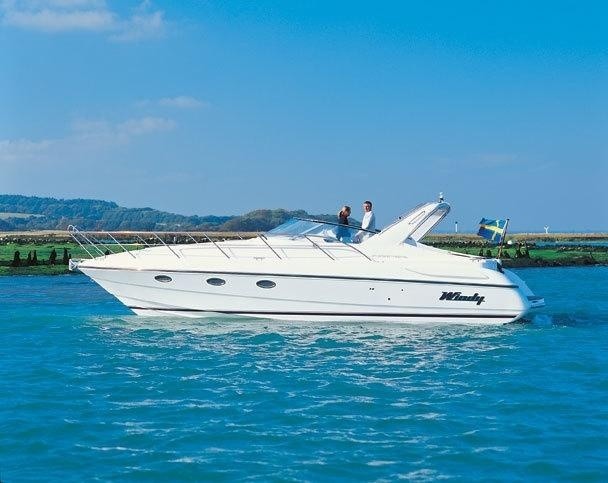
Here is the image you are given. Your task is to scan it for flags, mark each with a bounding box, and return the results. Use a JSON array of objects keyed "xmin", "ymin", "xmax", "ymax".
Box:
[{"xmin": 477, "ymin": 217, "xmax": 506, "ymax": 243}]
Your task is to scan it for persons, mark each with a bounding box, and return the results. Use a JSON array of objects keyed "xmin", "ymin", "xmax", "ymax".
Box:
[
  {"xmin": 337, "ymin": 201, "xmax": 376, "ymax": 246},
  {"xmin": 478, "ymin": 248, "xmax": 529, "ymax": 258},
  {"xmin": 11, "ymin": 247, "xmax": 72, "ymax": 267}
]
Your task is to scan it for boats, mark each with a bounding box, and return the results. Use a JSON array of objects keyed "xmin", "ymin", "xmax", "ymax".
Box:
[{"xmin": 68, "ymin": 192, "xmax": 544, "ymax": 326}]
